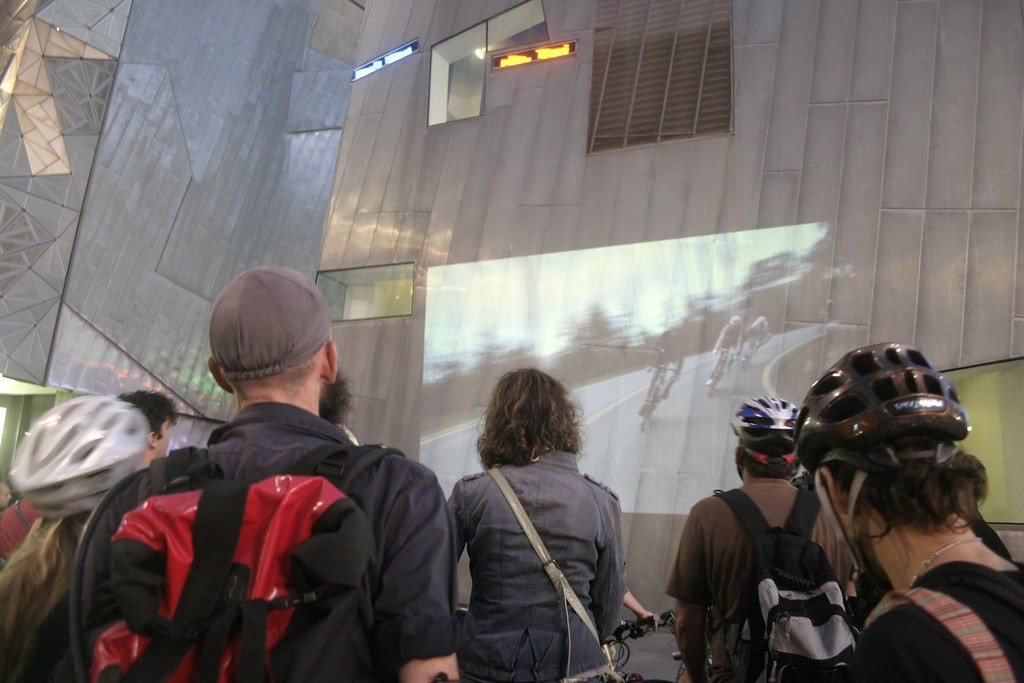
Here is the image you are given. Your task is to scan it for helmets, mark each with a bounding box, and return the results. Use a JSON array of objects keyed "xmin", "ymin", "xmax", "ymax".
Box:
[
  {"xmin": 732, "ymin": 395, "xmax": 801, "ymax": 451},
  {"xmin": 791, "ymin": 344, "xmax": 967, "ymax": 476},
  {"xmin": 7, "ymin": 395, "xmax": 153, "ymax": 516}
]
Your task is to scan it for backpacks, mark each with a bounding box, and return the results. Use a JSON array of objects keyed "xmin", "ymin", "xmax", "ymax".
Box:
[
  {"xmin": 705, "ymin": 489, "xmax": 855, "ymax": 683},
  {"xmin": 91, "ymin": 441, "xmax": 404, "ymax": 683}
]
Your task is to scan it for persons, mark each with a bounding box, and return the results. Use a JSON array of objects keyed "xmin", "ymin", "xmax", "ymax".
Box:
[
  {"xmin": 643, "ymin": 314, "xmax": 772, "ymax": 407},
  {"xmin": 664, "ymin": 397, "xmax": 857, "ymax": 683},
  {"xmin": 1, "ymin": 268, "xmax": 463, "ymax": 683},
  {"xmin": 448, "ymin": 369, "xmax": 623, "ymax": 683},
  {"xmin": 794, "ymin": 341, "xmax": 1024, "ymax": 682},
  {"xmin": 621, "ymin": 586, "xmax": 658, "ymax": 633}
]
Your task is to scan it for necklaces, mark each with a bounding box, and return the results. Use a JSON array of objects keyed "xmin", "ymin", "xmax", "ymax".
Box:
[{"xmin": 908, "ymin": 538, "xmax": 980, "ymax": 588}]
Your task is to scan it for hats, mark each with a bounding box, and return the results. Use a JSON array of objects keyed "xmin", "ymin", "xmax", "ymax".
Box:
[{"xmin": 209, "ymin": 267, "xmax": 331, "ymax": 380}]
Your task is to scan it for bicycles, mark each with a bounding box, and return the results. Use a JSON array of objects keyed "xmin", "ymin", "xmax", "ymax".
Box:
[
  {"xmin": 708, "ymin": 345, "xmax": 740, "ymax": 401},
  {"xmin": 638, "ymin": 357, "xmax": 678, "ymax": 432},
  {"xmin": 737, "ymin": 334, "xmax": 764, "ymax": 375},
  {"xmin": 602, "ymin": 606, "xmax": 713, "ymax": 683}
]
